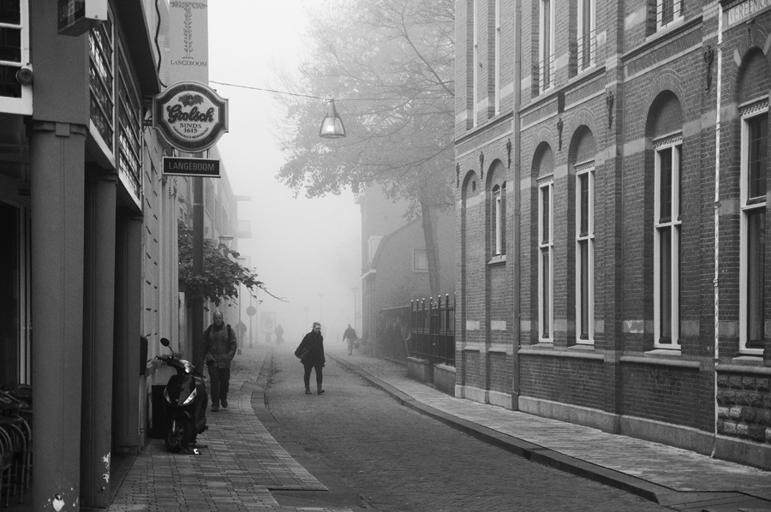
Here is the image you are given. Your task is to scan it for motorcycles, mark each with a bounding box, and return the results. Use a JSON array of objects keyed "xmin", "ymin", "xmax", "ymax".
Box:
[{"xmin": 153, "ymin": 337, "xmax": 208, "ymax": 454}]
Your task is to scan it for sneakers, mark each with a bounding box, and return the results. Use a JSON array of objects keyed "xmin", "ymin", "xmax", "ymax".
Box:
[
  {"xmin": 210, "ymin": 399, "xmax": 228, "ymax": 412},
  {"xmin": 305, "ymin": 388, "xmax": 325, "ymax": 394}
]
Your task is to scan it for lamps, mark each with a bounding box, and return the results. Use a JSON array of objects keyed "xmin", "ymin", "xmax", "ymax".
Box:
[{"xmin": 321, "ymin": 99, "xmax": 346, "ymax": 139}]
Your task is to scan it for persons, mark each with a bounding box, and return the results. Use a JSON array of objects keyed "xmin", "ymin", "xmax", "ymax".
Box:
[
  {"xmin": 198, "ymin": 311, "xmax": 237, "ymax": 412},
  {"xmin": 341, "ymin": 324, "xmax": 357, "ymax": 356},
  {"xmin": 294, "ymin": 322, "xmax": 326, "ymax": 394},
  {"xmin": 274, "ymin": 323, "xmax": 283, "ymax": 345},
  {"xmin": 392, "ymin": 314, "xmax": 411, "ymax": 364}
]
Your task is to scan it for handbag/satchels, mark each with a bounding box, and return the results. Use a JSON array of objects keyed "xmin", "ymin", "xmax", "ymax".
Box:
[{"xmin": 294, "ymin": 343, "xmax": 303, "ymax": 359}]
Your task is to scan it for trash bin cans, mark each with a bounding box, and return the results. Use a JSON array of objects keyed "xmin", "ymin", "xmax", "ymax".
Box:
[{"xmin": 152, "ymin": 385, "xmax": 166, "ymax": 439}]
